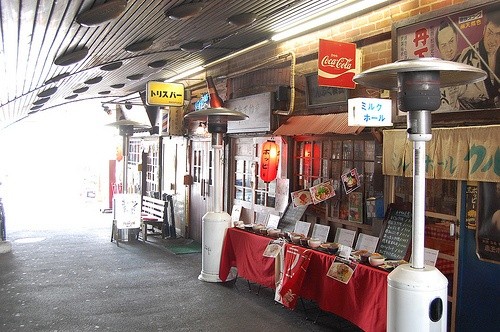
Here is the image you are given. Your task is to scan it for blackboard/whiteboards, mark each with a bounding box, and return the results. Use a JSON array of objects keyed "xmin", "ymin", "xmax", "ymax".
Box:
[
  {"xmin": 278, "ymin": 199, "xmax": 308, "ymax": 232},
  {"xmin": 375, "ymin": 203, "xmax": 412, "ymax": 260}
]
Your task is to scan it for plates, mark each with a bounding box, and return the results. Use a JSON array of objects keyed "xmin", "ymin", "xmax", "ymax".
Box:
[{"xmin": 381, "ymin": 259, "xmax": 408, "ymax": 268}]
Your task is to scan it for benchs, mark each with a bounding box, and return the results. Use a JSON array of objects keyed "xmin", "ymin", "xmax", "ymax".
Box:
[{"xmin": 139, "ymin": 195, "xmax": 169, "ymax": 243}]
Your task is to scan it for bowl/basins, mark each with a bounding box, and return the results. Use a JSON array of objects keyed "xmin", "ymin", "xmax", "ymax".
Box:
[{"xmin": 243, "ymin": 223, "xmax": 386, "ymax": 266}]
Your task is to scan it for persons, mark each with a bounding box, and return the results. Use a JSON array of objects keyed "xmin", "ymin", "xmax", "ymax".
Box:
[
  {"xmin": 436, "ymin": 19, "xmax": 464, "ymax": 112},
  {"xmin": 453, "ymin": 10, "xmax": 500, "ymax": 109}
]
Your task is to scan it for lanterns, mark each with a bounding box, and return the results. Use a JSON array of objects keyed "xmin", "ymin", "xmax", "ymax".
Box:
[
  {"xmin": 302, "ymin": 141, "xmax": 321, "ymax": 183},
  {"xmin": 259, "ymin": 139, "xmax": 281, "ymax": 183}
]
